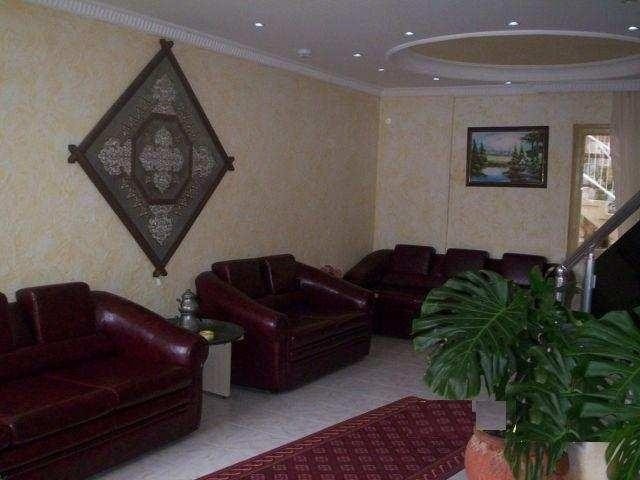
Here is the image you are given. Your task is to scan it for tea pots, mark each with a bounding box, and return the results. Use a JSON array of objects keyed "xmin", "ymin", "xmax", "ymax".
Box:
[
  {"xmin": 174, "ymin": 312, "xmax": 204, "ymax": 333},
  {"xmin": 177, "ymin": 289, "xmax": 200, "ymax": 320}
]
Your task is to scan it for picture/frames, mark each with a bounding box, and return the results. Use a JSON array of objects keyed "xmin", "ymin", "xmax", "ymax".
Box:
[
  {"xmin": 67, "ymin": 37, "xmax": 236, "ymax": 277},
  {"xmin": 465, "ymin": 126, "xmax": 548, "ymax": 188}
]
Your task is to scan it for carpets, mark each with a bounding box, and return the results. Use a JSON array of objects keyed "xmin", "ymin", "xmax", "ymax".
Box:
[{"xmin": 192, "ymin": 395, "xmax": 476, "ymax": 480}]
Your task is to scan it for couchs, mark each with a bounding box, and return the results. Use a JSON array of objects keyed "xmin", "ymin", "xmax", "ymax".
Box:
[
  {"xmin": 196, "ymin": 253, "xmax": 375, "ymax": 393},
  {"xmin": 0, "ymin": 282, "xmax": 209, "ymax": 480},
  {"xmin": 344, "ymin": 245, "xmax": 559, "ymax": 338}
]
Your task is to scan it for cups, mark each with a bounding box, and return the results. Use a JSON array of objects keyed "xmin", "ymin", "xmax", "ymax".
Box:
[{"xmin": 198, "ymin": 331, "xmax": 214, "ymax": 342}]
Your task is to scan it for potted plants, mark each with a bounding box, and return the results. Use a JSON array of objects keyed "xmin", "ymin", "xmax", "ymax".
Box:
[
  {"xmin": 505, "ymin": 309, "xmax": 640, "ymax": 480},
  {"xmin": 410, "ymin": 266, "xmax": 569, "ymax": 480}
]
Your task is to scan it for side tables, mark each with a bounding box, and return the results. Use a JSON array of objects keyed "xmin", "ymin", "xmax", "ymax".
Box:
[{"xmin": 168, "ymin": 318, "xmax": 244, "ymax": 398}]
